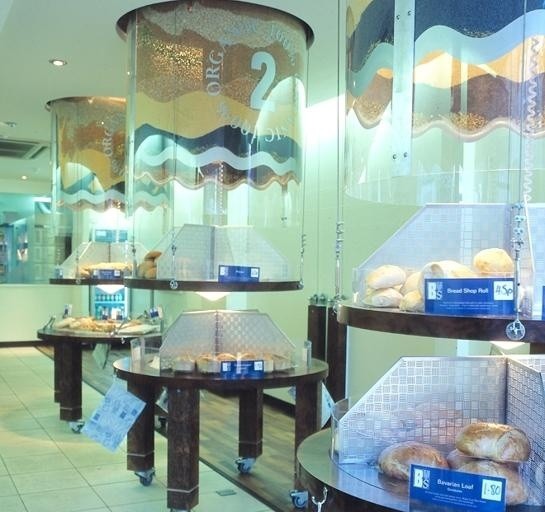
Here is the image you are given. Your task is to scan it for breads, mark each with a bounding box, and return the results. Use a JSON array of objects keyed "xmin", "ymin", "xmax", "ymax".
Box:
[
  {"xmin": 171, "ymin": 352, "xmax": 290, "ymax": 373},
  {"xmin": 362, "ymin": 246, "xmax": 515, "ymax": 313},
  {"xmin": 378, "ymin": 422, "xmax": 530, "ymax": 507},
  {"xmin": 55, "ymin": 251, "xmax": 162, "ymax": 332}
]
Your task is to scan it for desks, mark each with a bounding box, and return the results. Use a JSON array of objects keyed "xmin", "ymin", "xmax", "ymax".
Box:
[
  {"xmin": 297, "ymin": 428, "xmax": 544, "ymax": 511},
  {"xmin": 117, "ymin": 352, "xmax": 330, "ymax": 512},
  {"xmin": 37, "ymin": 321, "xmax": 165, "ymax": 435}
]
